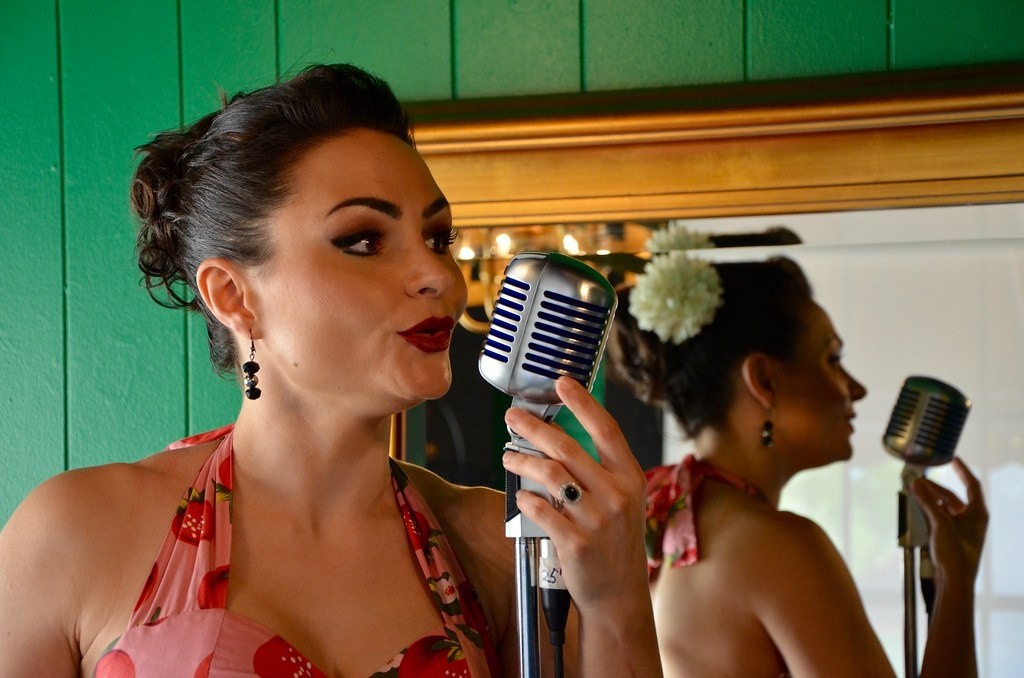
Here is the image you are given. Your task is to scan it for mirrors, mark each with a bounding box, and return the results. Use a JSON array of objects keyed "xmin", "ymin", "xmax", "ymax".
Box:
[{"xmin": 390, "ymin": 61, "xmax": 1024, "ymax": 678}]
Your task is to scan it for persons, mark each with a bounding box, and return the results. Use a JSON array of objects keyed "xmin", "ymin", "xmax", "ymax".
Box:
[
  {"xmin": 606, "ymin": 255, "xmax": 988, "ymax": 678},
  {"xmin": 0, "ymin": 63, "xmax": 662, "ymax": 678}
]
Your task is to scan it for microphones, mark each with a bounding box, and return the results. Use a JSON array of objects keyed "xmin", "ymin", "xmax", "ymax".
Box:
[
  {"xmin": 882, "ymin": 375, "xmax": 972, "ymax": 615},
  {"xmin": 478, "ymin": 250, "xmax": 619, "ymax": 644}
]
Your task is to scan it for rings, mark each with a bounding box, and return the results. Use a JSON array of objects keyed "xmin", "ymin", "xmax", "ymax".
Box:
[{"xmin": 559, "ymin": 481, "xmax": 583, "ymax": 505}]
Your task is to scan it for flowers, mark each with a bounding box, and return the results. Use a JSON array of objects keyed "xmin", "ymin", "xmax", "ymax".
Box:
[
  {"xmin": 628, "ymin": 250, "xmax": 723, "ymax": 344},
  {"xmin": 643, "ymin": 220, "xmax": 715, "ymax": 249}
]
